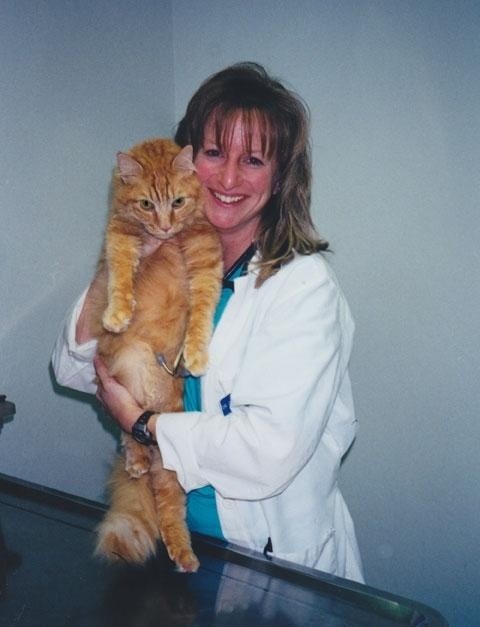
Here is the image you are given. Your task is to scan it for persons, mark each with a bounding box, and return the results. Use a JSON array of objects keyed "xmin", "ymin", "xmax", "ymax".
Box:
[
  {"xmin": 81, "ymin": 537, "xmax": 366, "ymax": 627},
  {"xmin": 49, "ymin": 62, "xmax": 372, "ymax": 586}
]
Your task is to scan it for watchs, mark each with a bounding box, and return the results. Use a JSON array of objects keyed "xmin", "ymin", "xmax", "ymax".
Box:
[{"xmin": 132, "ymin": 410, "xmax": 156, "ymax": 446}]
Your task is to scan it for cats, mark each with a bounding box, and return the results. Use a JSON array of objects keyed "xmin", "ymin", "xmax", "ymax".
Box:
[{"xmin": 94, "ymin": 137, "xmax": 225, "ymax": 575}]
